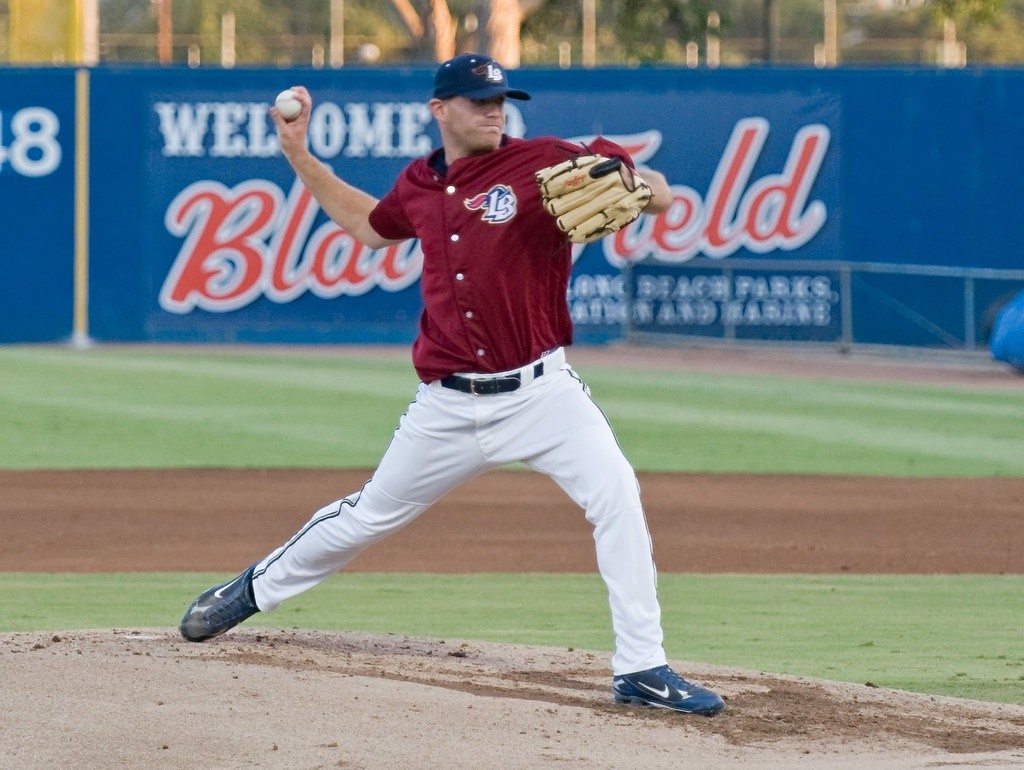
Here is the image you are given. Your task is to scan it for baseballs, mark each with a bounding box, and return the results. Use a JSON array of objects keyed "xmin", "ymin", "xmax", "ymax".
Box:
[{"xmin": 271, "ymin": 87, "xmax": 307, "ymax": 120}]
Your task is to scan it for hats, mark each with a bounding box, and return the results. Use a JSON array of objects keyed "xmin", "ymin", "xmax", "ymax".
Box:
[{"xmin": 433, "ymin": 52, "xmax": 531, "ymax": 101}]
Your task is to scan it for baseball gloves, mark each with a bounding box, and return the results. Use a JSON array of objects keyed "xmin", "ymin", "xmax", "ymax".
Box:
[{"xmin": 531, "ymin": 151, "xmax": 656, "ymax": 248}]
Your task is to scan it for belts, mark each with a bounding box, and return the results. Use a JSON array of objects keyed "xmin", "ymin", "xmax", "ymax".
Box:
[{"xmin": 440, "ymin": 362, "xmax": 543, "ymax": 396}]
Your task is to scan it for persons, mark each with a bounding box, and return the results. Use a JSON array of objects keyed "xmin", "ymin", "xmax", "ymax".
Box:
[{"xmin": 179, "ymin": 55, "xmax": 727, "ymax": 718}]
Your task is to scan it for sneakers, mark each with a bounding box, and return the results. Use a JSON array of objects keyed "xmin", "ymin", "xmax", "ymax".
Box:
[
  {"xmin": 612, "ymin": 665, "xmax": 725, "ymax": 716},
  {"xmin": 181, "ymin": 562, "xmax": 261, "ymax": 642}
]
